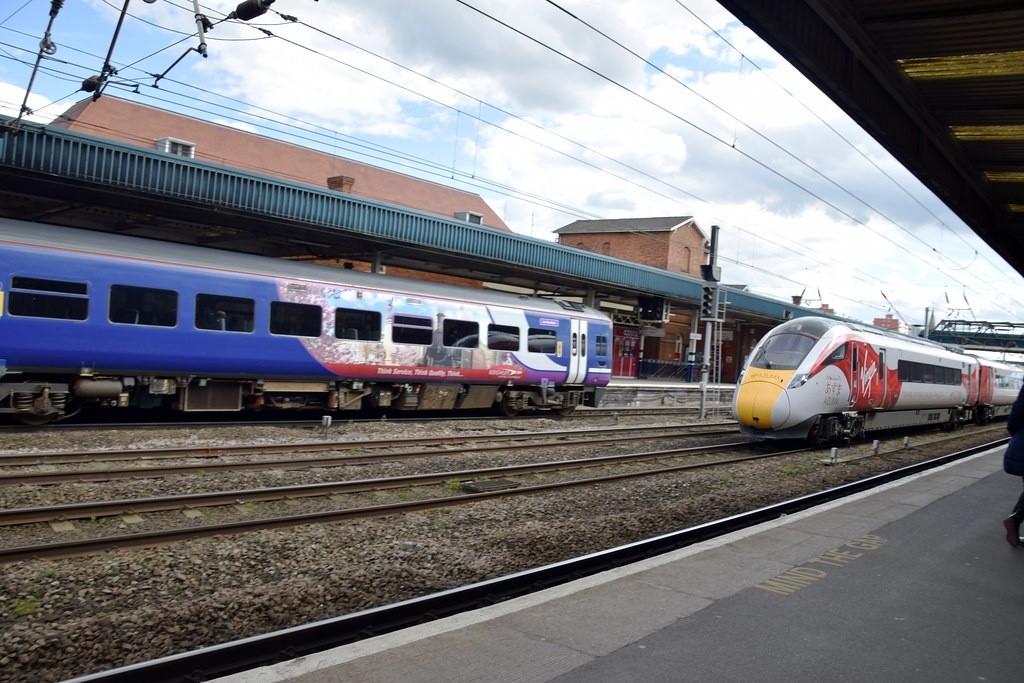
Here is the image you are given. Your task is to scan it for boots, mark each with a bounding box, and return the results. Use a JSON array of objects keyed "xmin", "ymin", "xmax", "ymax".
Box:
[{"xmin": 1003, "ymin": 513, "xmax": 1024, "ymax": 547}]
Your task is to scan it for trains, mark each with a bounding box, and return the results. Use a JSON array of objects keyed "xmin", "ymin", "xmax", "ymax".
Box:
[
  {"xmin": 731, "ymin": 316, "xmax": 1024, "ymax": 446},
  {"xmin": 1, "ymin": 217, "xmax": 613, "ymax": 419}
]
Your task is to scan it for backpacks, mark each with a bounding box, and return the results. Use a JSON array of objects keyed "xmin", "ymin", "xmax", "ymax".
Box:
[{"xmin": 1007, "ymin": 384, "xmax": 1024, "ymax": 436}]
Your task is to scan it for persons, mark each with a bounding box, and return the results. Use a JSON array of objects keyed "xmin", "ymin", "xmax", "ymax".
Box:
[{"xmin": 1004, "ymin": 382, "xmax": 1024, "ymax": 548}]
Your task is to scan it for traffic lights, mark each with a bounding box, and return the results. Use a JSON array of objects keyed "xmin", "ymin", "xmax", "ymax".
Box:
[
  {"xmin": 702, "ymin": 286, "xmax": 715, "ymax": 318},
  {"xmin": 700, "ymin": 265, "xmax": 720, "ymax": 281}
]
